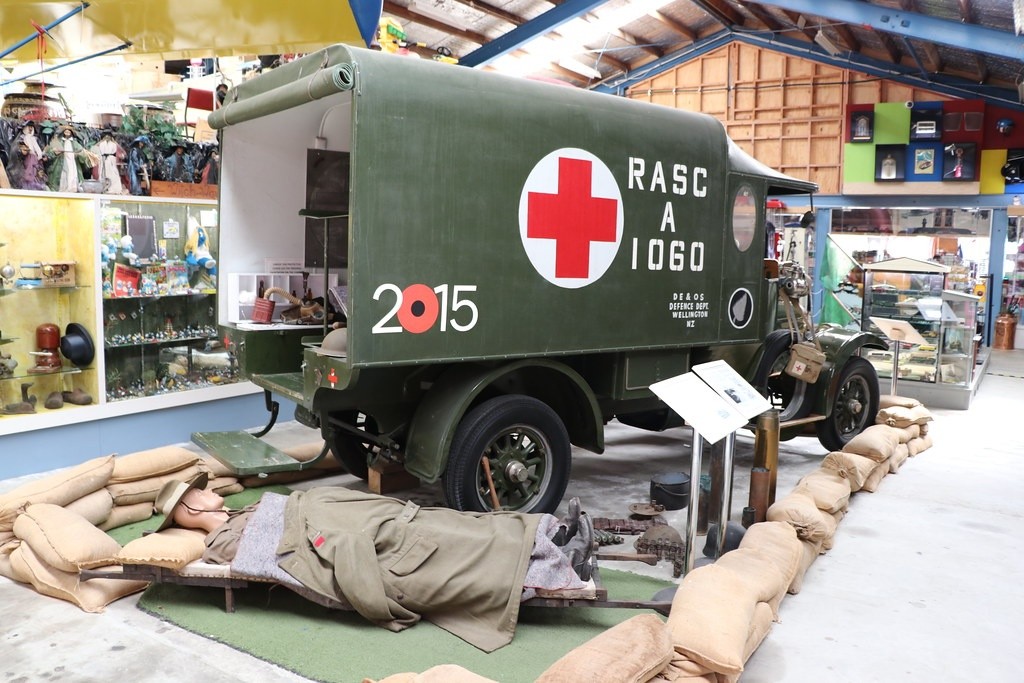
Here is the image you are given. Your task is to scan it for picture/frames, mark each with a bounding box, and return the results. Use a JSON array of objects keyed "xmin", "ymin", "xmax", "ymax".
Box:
[
  {"xmin": 875, "ymin": 144, "xmax": 905, "ymax": 182},
  {"xmin": 909, "ymin": 101, "xmax": 943, "ymax": 143},
  {"xmin": 851, "ymin": 111, "xmax": 873, "ymax": 143},
  {"xmin": 978, "ymin": 148, "xmax": 1024, "ymax": 194},
  {"xmin": 941, "ymin": 142, "xmax": 977, "ymax": 182},
  {"xmin": 905, "ymin": 143, "xmax": 944, "ymax": 182}
]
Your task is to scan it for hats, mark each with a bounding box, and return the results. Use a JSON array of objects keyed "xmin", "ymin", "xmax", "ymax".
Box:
[
  {"xmin": 60, "ymin": 323, "xmax": 95, "ymax": 366},
  {"xmin": 312, "ymin": 327, "xmax": 347, "ymax": 358},
  {"xmin": 155, "ymin": 472, "xmax": 208, "ymax": 533}
]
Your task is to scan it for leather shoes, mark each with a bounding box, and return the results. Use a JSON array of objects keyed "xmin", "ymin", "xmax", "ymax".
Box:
[
  {"xmin": 560, "ymin": 497, "xmax": 581, "ymax": 545},
  {"xmin": 562, "ymin": 513, "xmax": 594, "ymax": 581}
]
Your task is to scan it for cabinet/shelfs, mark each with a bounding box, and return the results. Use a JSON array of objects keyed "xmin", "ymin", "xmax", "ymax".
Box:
[
  {"xmin": 97, "ymin": 193, "xmax": 267, "ymax": 420},
  {"xmin": 0, "ymin": 194, "xmax": 102, "ymax": 436},
  {"xmin": 934, "ymin": 288, "xmax": 979, "ymax": 387},
  {"xmin": 854, "ymin": 257, "xmax": 950, "ymax": 385}
]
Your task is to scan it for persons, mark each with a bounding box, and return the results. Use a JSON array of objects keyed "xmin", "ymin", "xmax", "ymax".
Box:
[
  {"xmin": 0, "ymin": 53, "xmax": 313, "ymax": 199},
  {"xmin": 153, "ymin": 479, "xmax": 597, "ymax": 583}
]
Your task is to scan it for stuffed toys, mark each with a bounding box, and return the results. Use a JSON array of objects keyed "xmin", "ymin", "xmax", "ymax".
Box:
[{"xmin": 98, "ymin": 204, "xmax": 242, "ymax": 404}]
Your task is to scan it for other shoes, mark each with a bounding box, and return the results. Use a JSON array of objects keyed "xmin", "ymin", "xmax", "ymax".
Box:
[{"xmin": 44, "ymin": 388, "xmax": 93, "ymax": 409}]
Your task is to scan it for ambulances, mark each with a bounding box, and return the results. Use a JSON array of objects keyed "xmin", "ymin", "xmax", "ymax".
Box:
[{"xmin": 192, "ymin": 39, "xmax": 892, "ymax": 518}]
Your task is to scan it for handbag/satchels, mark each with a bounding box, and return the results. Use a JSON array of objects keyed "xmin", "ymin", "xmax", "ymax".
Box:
[{"xmin": 785, "ymin": 343, "xmax": 826, "ymax": 384}]
